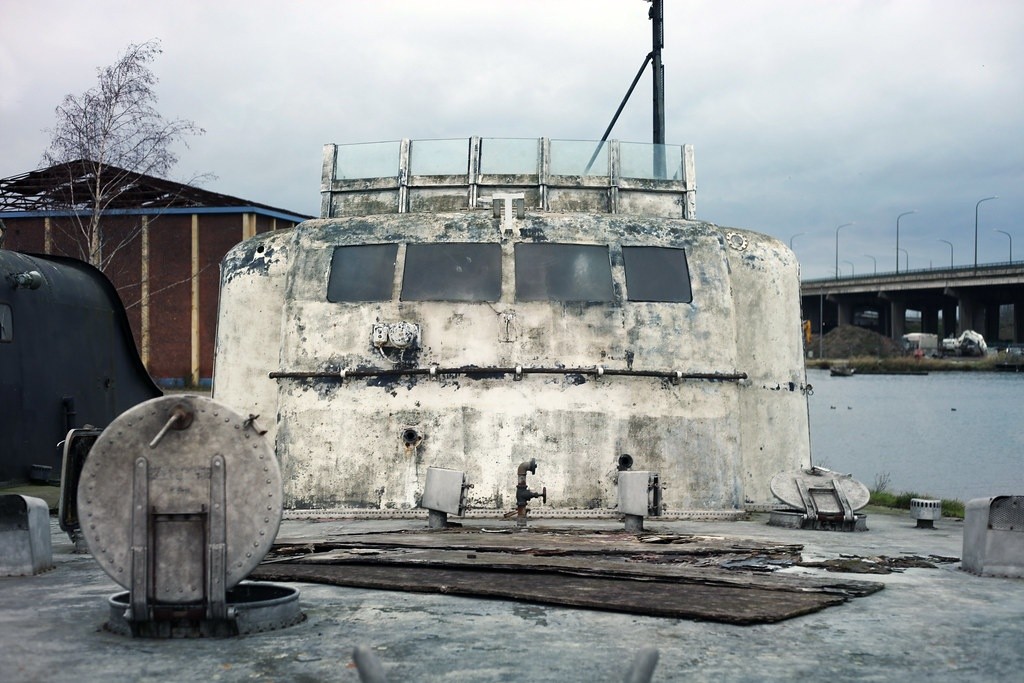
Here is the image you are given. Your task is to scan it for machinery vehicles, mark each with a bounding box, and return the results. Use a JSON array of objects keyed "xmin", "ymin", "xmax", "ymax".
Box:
[{"xmin": 799, "ymin": 319, "xmax": 813, "ymax": 359}]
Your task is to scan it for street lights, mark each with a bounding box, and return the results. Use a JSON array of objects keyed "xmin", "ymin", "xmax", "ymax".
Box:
[
  {"xmin": 786, "ymin": 233, "xmax": 804, "ymax": 251},
  {"xmin": 936, "ymin": 238, "xmax": 954, "ymax": 269},
  {"xmin": 844, "ymin": 259, "xmax": 855, "ymax": 278},
  {"xmin": 887, "ymin": 211, "xmax": 918, "ymax": 276},
  {"xmin": 866, "ymin": 254, "xmax": 877, "ymax": 276},
  {"xmin": 829, "ymin": 223, "xmax": 851, "ymax": 280},
  {"xmin": 893, "ymin": 247, "xmax": 911, "ymax": 272},
  {"xmin": 992, "ymin": 228, "xmax": 1014, "ymax": 264},
  {"xmin": 966, "ymin": 195, "xmax": 999, "ymax": 267}
]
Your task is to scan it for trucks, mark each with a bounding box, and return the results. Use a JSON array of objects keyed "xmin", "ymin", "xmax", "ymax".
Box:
[{"xmin": 900, "ymin": 332, "xmax": 938, "ymax": 359}]
